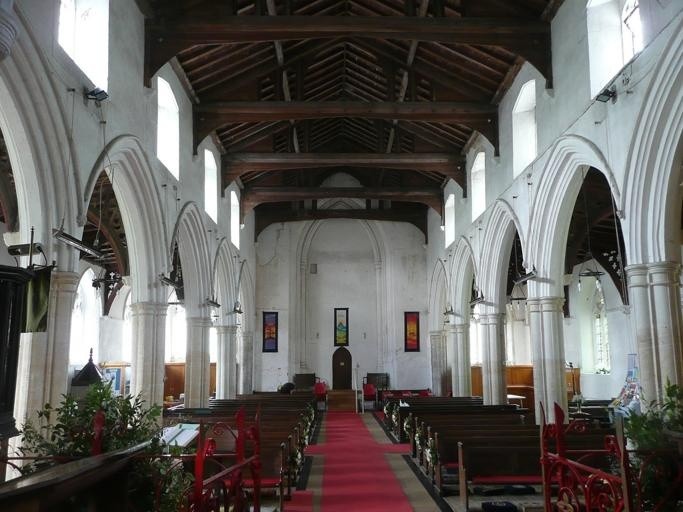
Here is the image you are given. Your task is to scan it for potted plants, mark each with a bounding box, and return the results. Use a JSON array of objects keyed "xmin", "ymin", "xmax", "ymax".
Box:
[{"xmin": 660, "ymin": 377, "xmax": 682, "ymax": 455}]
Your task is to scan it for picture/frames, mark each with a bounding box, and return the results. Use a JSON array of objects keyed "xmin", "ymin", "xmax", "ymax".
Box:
[
  {"xmin": 261, "ymin": 311, "xmax": 280, "ymax": 353},
  {"xmin": 332, "ymin": 307, "xmax": 351, "ymax": 348},
  {"xmin": 403, "ymin": 311, "xmax": 422, "ymax": 353}
]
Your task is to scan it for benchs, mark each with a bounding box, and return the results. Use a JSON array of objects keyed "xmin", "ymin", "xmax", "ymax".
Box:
[
  {"xmin": 381, "ymin": 394, "xmax": 621, "ymax": 511},
  {"xmin": 163, "ymin": 389, "xmax": 320, "ymax": 511}
]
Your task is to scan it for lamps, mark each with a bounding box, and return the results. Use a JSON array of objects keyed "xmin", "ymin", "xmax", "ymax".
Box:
[
  {"xmin": 49, "ymin": 225, "xmax": 109, "ymax": 267},
  {"xmin": 594, "ymin": 83, "xmax": 620, "ymax": 107},
  {"xmin": 443, "ymin": 295, "xmax": 487, "ymax": 316},
  {"xmin": 577, "ymin": 180, "xmax": 606, "ymax": 293},
  {"xmin": 511, "ymin": 235, "xmax": 529, "ymax": 311},
  {"xmin": 203, "ymin": 296, "xmax": 247, "ymax": 316},
  {"xmin": 155, "ymin": 271, "xmax": 185, "ymax": 291},
  {"xmin": 512, "ymin": 268, "xmax": 539, "ymax": 289},
  {"xmin": 78, "ymin": 87, "xmax": 111, "ymax": 113}
]
